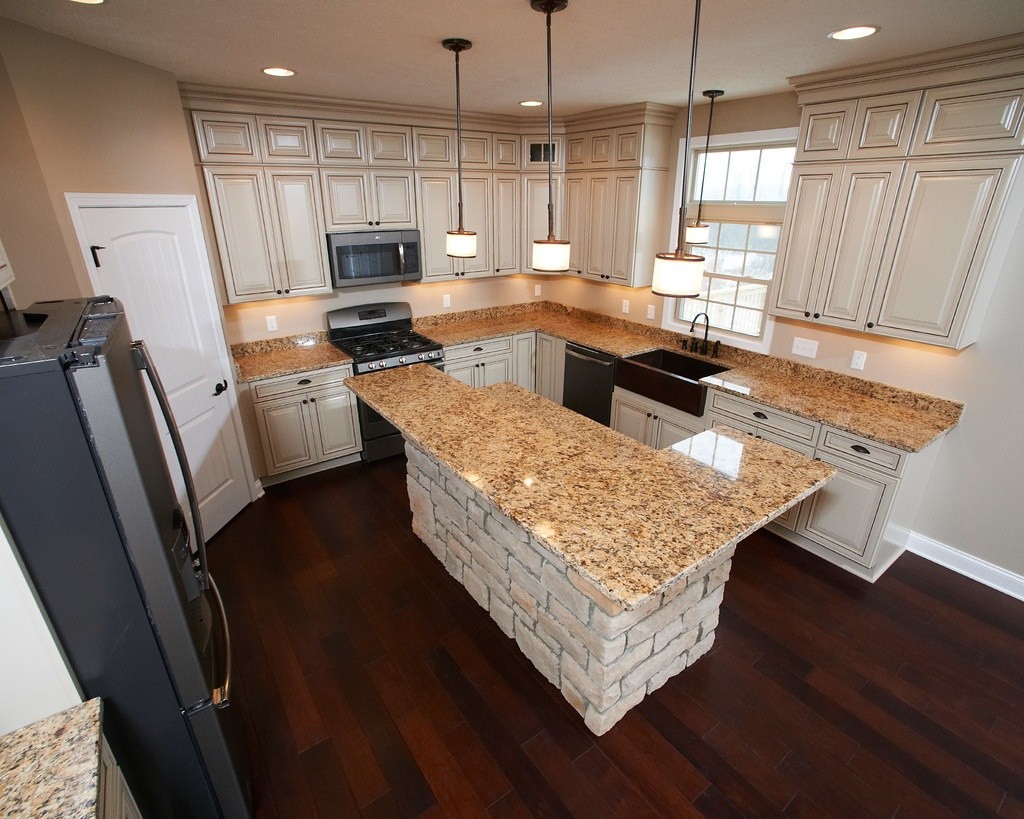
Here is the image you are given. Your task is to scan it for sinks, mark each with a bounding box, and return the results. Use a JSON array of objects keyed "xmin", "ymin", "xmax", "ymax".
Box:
[{"xmin": 614, "ymin": 348, "xmax": 731, "ymax": 417}]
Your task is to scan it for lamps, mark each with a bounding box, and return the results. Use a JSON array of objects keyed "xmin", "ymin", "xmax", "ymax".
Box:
[
  {"xmin": 531, "ymin": 0, "xmax": 570, "ymax": 272},
  {"xmin": 686, "ymin": 89, "xmax": 724, "ymax": 244},
  {"xmin": 652, "ymin": 0, "xmax": 706, "ymax": 297},
  {"xmin": 442, "ymin": 38, "xmax": 477, "ymax": 258}
]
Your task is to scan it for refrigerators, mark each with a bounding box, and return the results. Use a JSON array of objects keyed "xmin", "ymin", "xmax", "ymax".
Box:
[{"xmin": 0, "ymin": 296, "xmax": 254, "ymax": 819}]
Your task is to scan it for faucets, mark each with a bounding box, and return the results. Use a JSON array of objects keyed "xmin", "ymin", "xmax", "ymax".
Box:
[{"xmin": 689, "ymin": 312, "xmax": 710, "ymax": 355}]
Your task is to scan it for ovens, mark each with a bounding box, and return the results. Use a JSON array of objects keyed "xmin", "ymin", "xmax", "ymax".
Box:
[{"xmin": 354, "ymin": 359, "xmax": 446, "ymax": 464}]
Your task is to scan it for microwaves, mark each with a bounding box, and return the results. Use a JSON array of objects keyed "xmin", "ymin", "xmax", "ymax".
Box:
[{"xmin": 327, "ymin": 230, "xmax": 423, "ymax": 289}]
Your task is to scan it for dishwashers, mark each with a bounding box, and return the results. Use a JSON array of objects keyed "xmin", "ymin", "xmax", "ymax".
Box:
[{"xmin": 562, "ymin": 341, "xmax": 617, "ymax": 428}]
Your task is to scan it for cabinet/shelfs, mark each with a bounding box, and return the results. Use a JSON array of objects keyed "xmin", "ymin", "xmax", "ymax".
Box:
[
  {"xmin": 609, "ymin": 393, "xmax": 705, "ymax": 451},
  {"xmin": 767, "ymin": 32, "xmax": 1024, "ymax": 349},
  {"xmin": 178, "ymin": 82, "xmax": 680, "ymax": 309},
  {"xmin": 443, "ymin": 332, "xmax": 567, "ymax": 405},
  {"xmin": 705, "ymin": 387, "xmax": 943, "ymax": 584},
  {"xmin": 250, "ymin": 363, "xmax": 363, "ymax": 476},
  {"xmin": 98, "ymin": 731, "xmax": 141, "ymax": 819}
]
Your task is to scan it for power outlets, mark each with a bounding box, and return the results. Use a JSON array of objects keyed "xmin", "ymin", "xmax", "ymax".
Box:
[
  {"xmin": 266, "ymin": 316, "xmax": 277, "ymax": 331},
  {"xmin": 850, "ymin": 350, "xmax": 867, "ymax": 371},
  {"xmin": 792, "ymin": 336, "xmax": 818, "ymax": 359}
]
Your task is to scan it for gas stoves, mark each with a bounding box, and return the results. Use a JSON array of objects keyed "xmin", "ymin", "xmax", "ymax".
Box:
[{"xmin": 326, "ymin": 303, "xmax": 444, "ymax": 374}]
